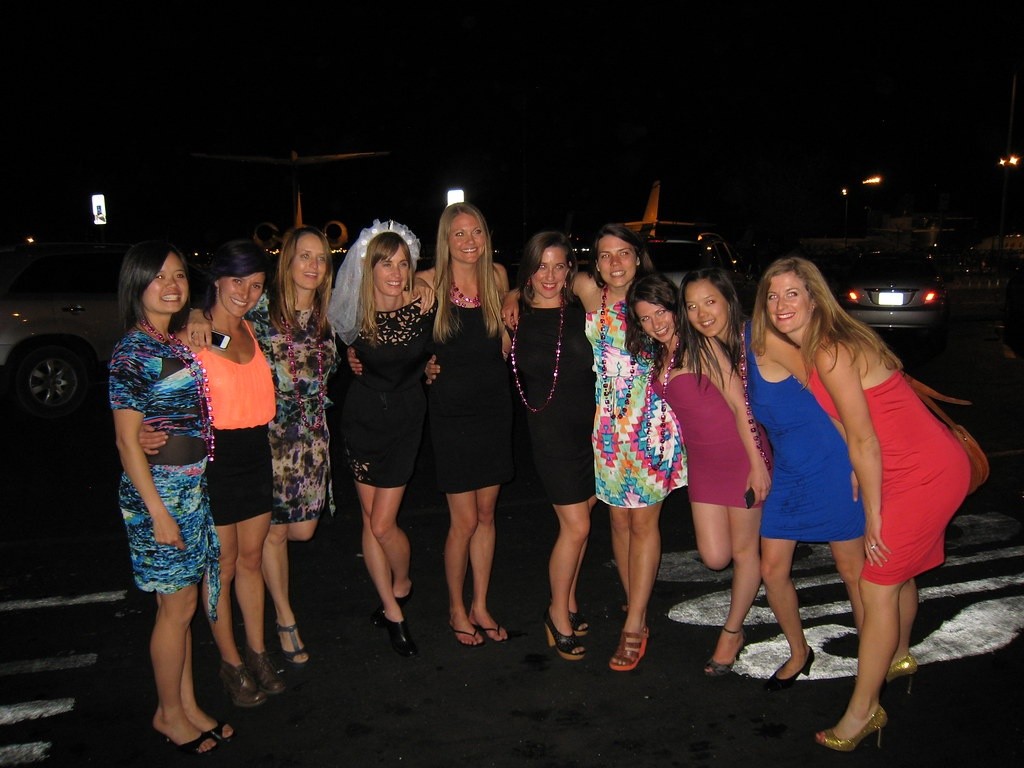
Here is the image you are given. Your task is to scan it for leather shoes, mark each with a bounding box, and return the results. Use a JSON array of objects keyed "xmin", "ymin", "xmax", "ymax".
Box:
[
  {"xmin": 218, "ymin": 662, "xmax": 267, "ymax": 706},
  {"xmin": 245, "ymin": 647, "xmax": 287, "ymax": 692},
  {"xmin": 393, "ymin": 581, "xmax": 412, "ymax": 604},
  {"xmin": 383, "ymin": 611, "xmax": 417, "ymax": 656}
]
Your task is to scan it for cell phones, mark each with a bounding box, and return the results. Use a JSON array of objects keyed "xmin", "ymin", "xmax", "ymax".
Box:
[
  {"xmin": 211, "ymin": 330, "xmax": 231, "ymax": 351},
  {"xmin": 743, "ymin": 487, "xmax": 755, "ymax": 509}
]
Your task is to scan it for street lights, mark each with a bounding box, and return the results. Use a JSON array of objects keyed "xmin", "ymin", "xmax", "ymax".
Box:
[
  {"xmin": 997, "ymin": 155, "xmax": 1019, "ymax": 260},
  {"xmin": 842, "ymin": 189, "xmax": 849, "ymax": 248}
]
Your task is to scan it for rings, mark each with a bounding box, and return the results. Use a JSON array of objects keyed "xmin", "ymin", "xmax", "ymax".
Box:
[
  {"xmin": 192, "ymin": 332, "xmax": 198, "ymax": 337},
  {"xmin": 502, "ymin": 318, "xmax": 505, "ymax": 321},
  {"xmin": 871, "ymin": 544, "xmax": 878, "ymax": 550}
]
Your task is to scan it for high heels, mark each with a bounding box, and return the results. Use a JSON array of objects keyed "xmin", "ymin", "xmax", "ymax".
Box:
[
  {"xmin": 569, "ymin": 610, "xmax": 588, "ymax": 636},
  {"xmin": 609, "ymin": 626, "xmax": 650, "ymax": 671},
  {"xmin": 152, "ymin": 724, "xmax": 218, "ymax": 755},
  {"xmin": 815, "ymin": 705, "xmax": 887, "ymax": 752},
  {"xmin": 543, "ymin": 605, "xmax": 585, "ymax": 660},
  {"xmin": 886, "ymin": 654, "xmax": 917, "ymax": 693},
  {"xmin": 277, "ymin": 622, "xmax": 310, "ymax": 670},
  {"xmin": 203, "ymin": 719, "xmax": 236, "ymax": 742},
  {"xmin": 767, "ymin": 646, "xmax": 813, "ymax": 691},
  {"xmin": 704, "ymin": 629, "xmax": 746, "ymax": 676}
]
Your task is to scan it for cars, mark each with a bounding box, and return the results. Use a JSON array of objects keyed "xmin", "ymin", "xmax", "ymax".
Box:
[{"xmin": 838, "ymin": 255, "xmax": 952, "ymax": 338}]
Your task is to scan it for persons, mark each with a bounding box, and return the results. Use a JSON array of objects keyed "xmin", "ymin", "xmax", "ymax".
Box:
[
  {"xmin": 500, "ymin": 223, "xmax": 688, "ymax": 670},
  {"xmin": 412, "ymin": 202, "xmax": 510, "ymax": 647},
  {"xmin": 107, "ymin": 241, "xmax": 238, "ymax": 756},
  {"xmin": 425, "ymin": 232, "xmax": 597, "ymax": 660},
  {"xmin": 625, "ymin": 274, "xmax": 774, "ymax": 676},
  {"xmin": 677, "ymin": 267, "xmax": 866, "ymax": 691},
  {"xmin": 139, "ymin": 240, "xmax": 287, "ymax": 706},
  {"xmin": 750, "ymin": 257, "xmax": 971, "ymax": 751},
  {"xmin": 326, "ymin": 219, "xmax": 430, "ymax": 657},
  {"xmin": 187, "ymin": 227, "xmax": 341, "ymax": 665}
]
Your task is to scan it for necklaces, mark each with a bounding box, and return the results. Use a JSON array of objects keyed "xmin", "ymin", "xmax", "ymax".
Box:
[
  {"xmin": 601, "ymin": 285, "xmax": 636, "ymax": 419},
  {"xmin": 647, "ymin": 342, "xmax": 679, "ymax": 471},
  {"xmin": 741, "ymin": 322, "xmax": 771, "ymax": 469},
  {"xmin": 450, "ymin": 281, "xmax": 480, "ymax": 308},
  {"xmin": 510, "ymin": 289, "xmax": 564, "ymax": 413},
  {"xmin": 141, "ymin": 319, "xmax": 216, "ymax": 461},
  {"xmin": 285, "ymin": 320, "xmax": 324, "ymax": 430}
]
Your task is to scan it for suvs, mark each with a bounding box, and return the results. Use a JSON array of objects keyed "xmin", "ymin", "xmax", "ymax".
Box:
[
  {"xmin": 1, "ymin": 242, "xmax": 214, "ymax": 423},
  {"xmin": 640, "ymin": 231, "xmax": 760, "ymax": 313}
]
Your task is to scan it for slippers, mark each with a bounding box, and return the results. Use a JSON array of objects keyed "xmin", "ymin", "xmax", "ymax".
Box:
[
  {"xmin": 448, "ymin": 621, "xmax": 485, "ymax": 647},
  {"xmin": 469, "ymin": 619, "xmax": 508, "ymax": 644}
]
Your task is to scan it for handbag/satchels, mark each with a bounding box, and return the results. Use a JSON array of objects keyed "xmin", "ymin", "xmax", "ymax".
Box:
[{"xmin": 953, "ymin": 424, "xmax": 989, "ymax": 492}]
